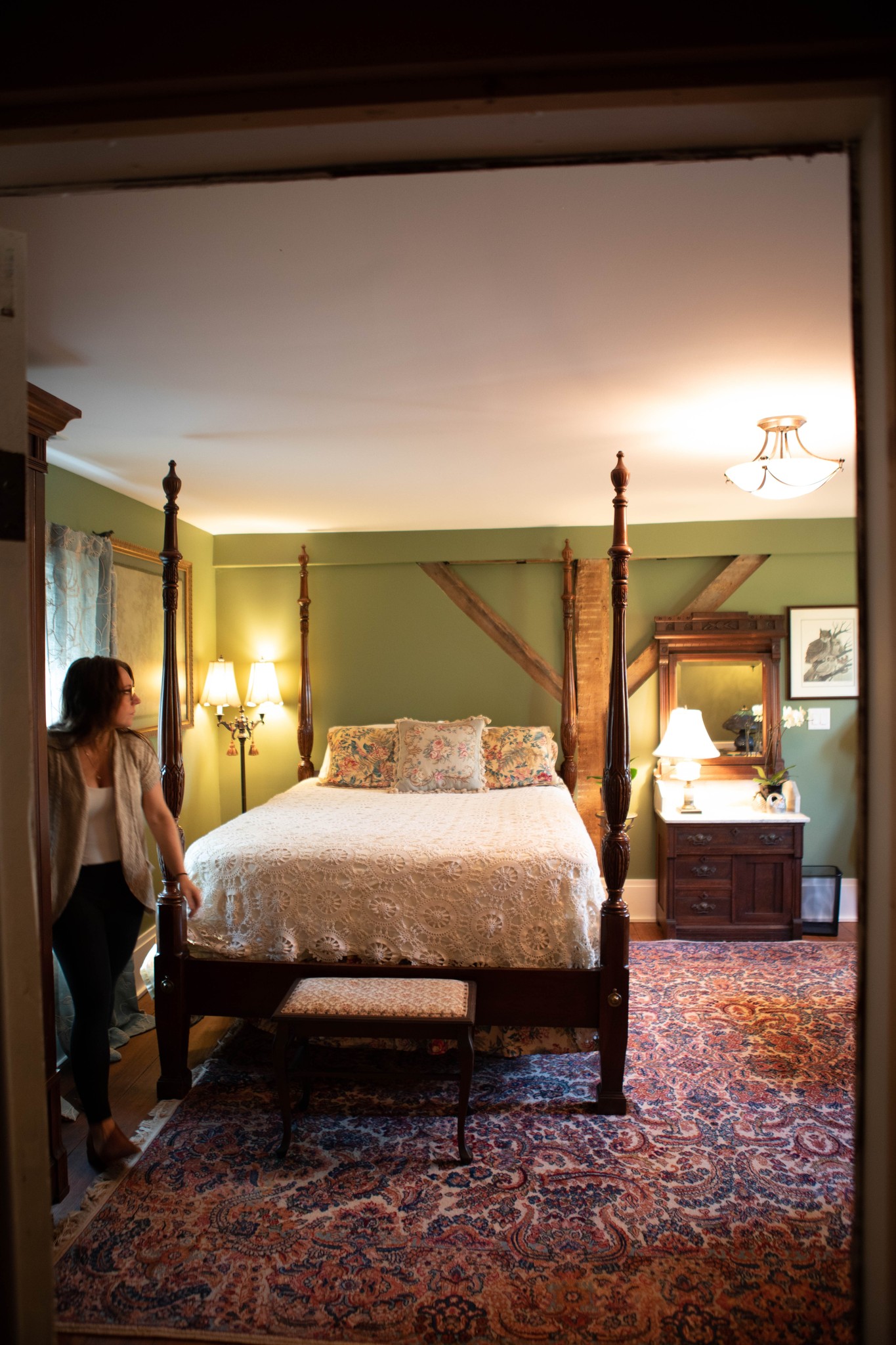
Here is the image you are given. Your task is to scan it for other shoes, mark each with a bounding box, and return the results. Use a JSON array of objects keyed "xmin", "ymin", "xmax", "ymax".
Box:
[
  {"xmin": 94, "ymin": 1120, "xmax": 140, "ymax": 1159},
  {"xmin": 86, "ymin": 1137, "xmax": 128, "ymax": 1175}
]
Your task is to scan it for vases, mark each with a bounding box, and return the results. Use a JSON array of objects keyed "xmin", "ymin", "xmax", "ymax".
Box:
[{"xmin": 757, "ymin": 783, "xmax": 783, "ymax": 801}]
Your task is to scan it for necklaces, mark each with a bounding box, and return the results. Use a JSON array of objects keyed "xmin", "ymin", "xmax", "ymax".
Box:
[{"xmin": 82, "ymin": 743, "xmax": 109, "ymax": 779}]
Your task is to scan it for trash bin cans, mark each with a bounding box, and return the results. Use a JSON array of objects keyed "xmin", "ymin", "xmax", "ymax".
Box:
[{"xmin": 801, "ymin": 865, "xmax": 844, "ymax": 936}]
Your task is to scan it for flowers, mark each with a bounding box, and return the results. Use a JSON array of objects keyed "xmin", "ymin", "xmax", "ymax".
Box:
[
  {"xmin": 751, "ymin": 703, "xmax": 815, "ymax": 786},
  {"xmin": 744, "ymin": 704, "xmax": 763, "ymax": 756}
]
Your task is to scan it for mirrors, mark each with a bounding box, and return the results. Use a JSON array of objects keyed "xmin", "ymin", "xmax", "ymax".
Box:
[{"xmin": 651, "ymin": 611, "xmax": 792, "ymax": 787}]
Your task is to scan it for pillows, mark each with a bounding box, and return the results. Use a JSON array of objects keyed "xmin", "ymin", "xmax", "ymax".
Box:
[
  {"xmin": 314, "ymin": 726, "xmax": 398, "ymax": 788},
  {"xmin": 393, "ymin": 715, "xmax": 493, "ymax": 795},
  {"xmin": 481, "ymin": 726, "xmax": 564, "ymax": 790}
]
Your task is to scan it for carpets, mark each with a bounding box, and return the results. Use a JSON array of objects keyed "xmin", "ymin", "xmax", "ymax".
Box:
[{"xmin": 46, "ymin": 939, "xmax": 861, "ymax": 1345}]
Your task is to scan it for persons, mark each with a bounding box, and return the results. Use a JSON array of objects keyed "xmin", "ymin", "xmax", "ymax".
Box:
[{"xmin": 45, "ymin": 655, "xmax": 201, "ymax": 1159}]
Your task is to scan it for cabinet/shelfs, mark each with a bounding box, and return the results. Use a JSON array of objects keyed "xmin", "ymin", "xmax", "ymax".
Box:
[{"xmin": 655, "ymin": 807, "xmax": 811, "ymax": 941}]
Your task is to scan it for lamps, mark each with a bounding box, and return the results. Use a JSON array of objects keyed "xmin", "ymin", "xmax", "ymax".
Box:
[
  {"xmin": 723, "ymin": 705, "xmax": 762, "ymax": 752},
  {"xmin": 195, "ymin": 656, "xmax": 286, "ymax": 816},
  {"xmin": 650, "ymin": 706, "xmax": 723, "ymax": 815},
  {"xmin": 722, "ymin": 415, "xmax": 850, "ymax": 503}
]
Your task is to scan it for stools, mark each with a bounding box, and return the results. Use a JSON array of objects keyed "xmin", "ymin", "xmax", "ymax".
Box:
[{"xmin": 269, "ymin": 977, "xmax": 476, "ymax": 1166}]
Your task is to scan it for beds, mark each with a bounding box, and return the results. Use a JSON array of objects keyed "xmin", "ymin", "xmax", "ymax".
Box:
[{"xmin": 152, "ymin": 450, "xmax": 634, "ymax": 1117}]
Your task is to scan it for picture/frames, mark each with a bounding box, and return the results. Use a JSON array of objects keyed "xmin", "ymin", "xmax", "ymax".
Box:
[
  {"xmin": 785, "ymin": 604, "xmax": 863, "ymax": 702},
  {"xmin": 107, "ymin": 536, "xmax": 193, "ymax": 740}
]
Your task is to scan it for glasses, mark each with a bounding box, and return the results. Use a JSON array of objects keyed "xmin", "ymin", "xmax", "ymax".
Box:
[{"xmin": 120, "ymin": 687, "xmax": 136, "ymax": 700}]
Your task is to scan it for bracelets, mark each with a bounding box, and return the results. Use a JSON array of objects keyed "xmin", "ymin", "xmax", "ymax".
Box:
[{"xmin": 175, "ymin": 873, "xmax": 187, "ymax": 880}]
